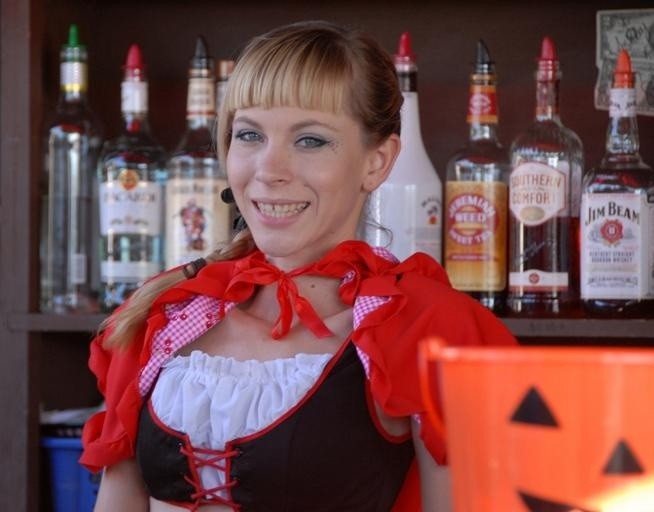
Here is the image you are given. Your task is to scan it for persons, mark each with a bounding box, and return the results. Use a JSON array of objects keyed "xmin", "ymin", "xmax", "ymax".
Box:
[{"xmin": 93, "ymin": 20, "xmax": 518, "ymax": 512}]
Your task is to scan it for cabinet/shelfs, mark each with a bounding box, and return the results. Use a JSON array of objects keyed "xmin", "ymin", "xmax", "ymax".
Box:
[{"xmin": 0, "ymin": 0, "xmax": 654, "ymax": 512}]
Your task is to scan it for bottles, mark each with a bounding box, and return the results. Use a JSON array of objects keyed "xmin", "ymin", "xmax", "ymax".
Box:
[
  {"xmin": 159, "ymin": 40, "xmax": 233, "ymax": 277},
  {"xmin": 580, "ymin": 48, "xmax": 654, "ymax": 322},
  {"xmin": 91, "ymin": 42, "xmax": 163, "ymax": 314},
  {"xmin": 36, "ymin": 19, "xmax": 101, "ymax": 317},
  {"xmin": 506, "ymin": 35, "xmax": 584, "ymax": 320},
  {"xmin": 215, "ymin": 57, "xmax": 236, "ymax": 126},
  {"xmin": 440, "ymin": 38, "xmax": 509, "ymax": 318},
  {"xmin": 361, "ymin": 26, "xmax": 441, "ymax": 268}
]
